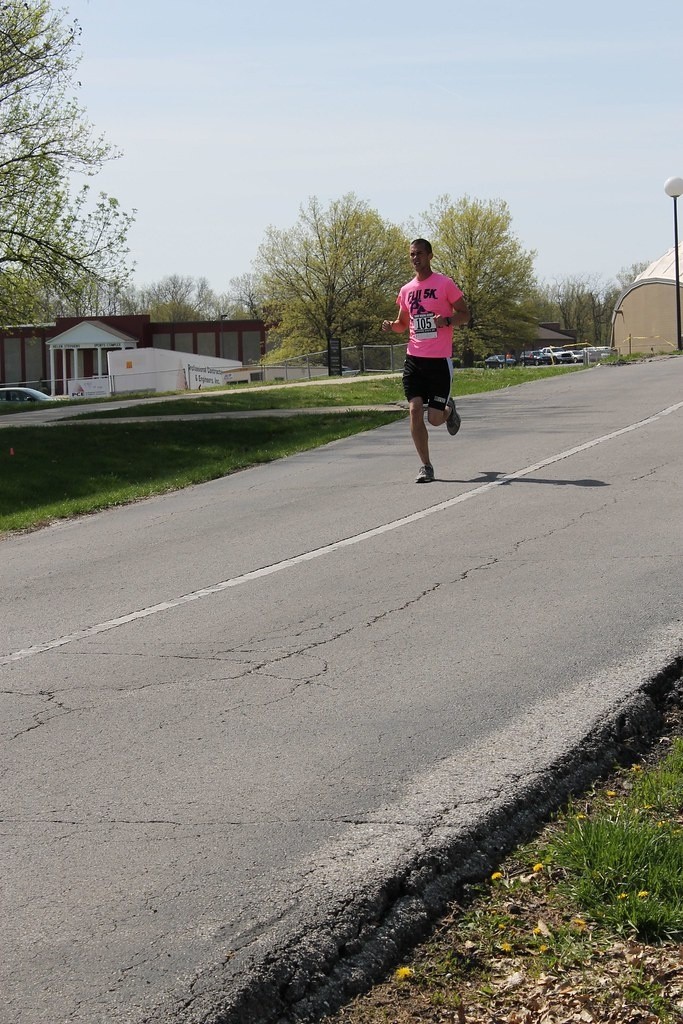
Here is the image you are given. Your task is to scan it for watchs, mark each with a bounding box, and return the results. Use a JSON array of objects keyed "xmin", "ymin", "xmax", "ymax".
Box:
[{"xmin": 445, "ymin": 317, "xmax": 451, "ymax": 327}]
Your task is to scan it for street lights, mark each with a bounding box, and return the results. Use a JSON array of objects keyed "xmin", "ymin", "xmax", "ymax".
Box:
[
  {"xmin": 220, "ymin": 314, "xmax": 228, "ymax": 358},
  {"xmin": 663, "ymin": 175, "xmax": 683, "ymax": 350}
]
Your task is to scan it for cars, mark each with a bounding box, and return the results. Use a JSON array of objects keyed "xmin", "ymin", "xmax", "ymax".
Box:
[
  {"xmin": 519, "ymin": 345, "xmax": 610, "ymax": 368},
  {"xmin": 0, "ymin": 386, "xmax": 53, "ymax": 402},
  {"xmin": 485, "ymin": 355, "xmax": 517, "ymax": 369}
]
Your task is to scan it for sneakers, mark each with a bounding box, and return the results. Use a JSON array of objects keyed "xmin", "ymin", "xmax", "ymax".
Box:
[
  {"xmin": 445, "ymin": 397, "xmax": 461, "ymax": 436},
  {"xmin": 413, "ymin": 464, "xmax": 435, "ymax": 482}
]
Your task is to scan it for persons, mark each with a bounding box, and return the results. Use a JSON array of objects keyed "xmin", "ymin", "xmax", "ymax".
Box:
[{"xmin": 382, "ymin": 238, "xmax": 470, "ymax": 482}]
